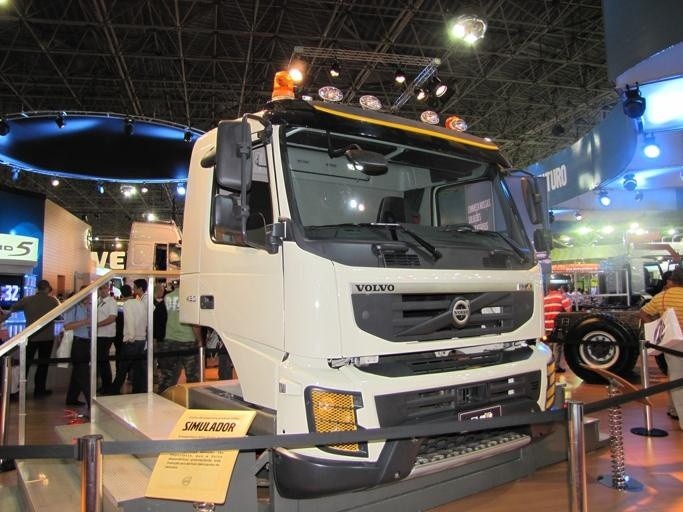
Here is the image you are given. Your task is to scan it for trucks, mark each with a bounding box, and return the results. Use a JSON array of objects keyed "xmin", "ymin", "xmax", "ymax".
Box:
[
  {"xmin": 167, "ymin": 58, "xmax": 559, "ymax": 504},
  {"xmin": 118, "ymin": 217, "xmax": 183, "ymax": 296}
]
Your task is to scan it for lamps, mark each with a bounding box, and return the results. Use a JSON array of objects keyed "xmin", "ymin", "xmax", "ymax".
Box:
[
  {"xmin": 0, "ymin": 115, "xmax": 192, "ymax": 142},
  {"xmin": 329, "ymin": 57, "xmax": 447, "ymax": 100},
  {"xmin": 573, "ymin": 83, "xmax": 662, "ymax": 222}
]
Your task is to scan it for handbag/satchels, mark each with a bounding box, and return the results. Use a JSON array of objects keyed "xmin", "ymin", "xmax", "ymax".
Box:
[{"xmin": 644, "ymin": 308, "xmax": 683, "ymax": 356}]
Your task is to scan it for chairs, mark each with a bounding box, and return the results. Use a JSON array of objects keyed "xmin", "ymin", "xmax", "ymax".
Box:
[
  {"xmin": 376, "ymin": 196, "xmax": 414, "ymax": 222},
  {"xmin": 233, "ymin": 182, "xmax": 271, "ymax": 229}
]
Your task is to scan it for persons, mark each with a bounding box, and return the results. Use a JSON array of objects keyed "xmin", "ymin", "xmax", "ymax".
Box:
[
  {"xmin": 0, "ymin": 292, "xmax": 13, "ymax": 399},
  {"xmin": 559, "ymin": 284, "xmax": 573, "ymax": 311},
  {"xmin": 9, "ymin": 279, "xmax": 58, "ymax": 402},
  {"xmin": 635, "ymin": 262, "xmax": 683, "ymax": 435},
  {"xmin": 58, "ymin": 276, "xmax": 233, "ymax": 417},
  {"xmin": 542, "ymin": 283, "xmax": 573, "ymax": 374},
  {"xmin": 567, "ymin": 286, "xmax": 574, "ymax": 295},
  {"xmin": 573, "ymin": 287, "xmax": 582, "ymax": 312}
]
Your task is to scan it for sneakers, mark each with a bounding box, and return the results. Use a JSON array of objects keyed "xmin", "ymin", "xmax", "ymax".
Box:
[
  {"xmin": 67, "ymin": 399, "xmax": 86, "ymax": 406},
  {"xmin": 666, "ymin": 408, "xmax": 678, "ymax": 419},
  {"xmin": 35, "ymin": 389, "xmax": 52, "ymax": 399},
  {"xmin": 556, "ymin": 367, "xmax": 566, "ymax": 372},
  {"xmin": 10, "ymin": 392, "xmax": 27, "ymax": 400}
]
